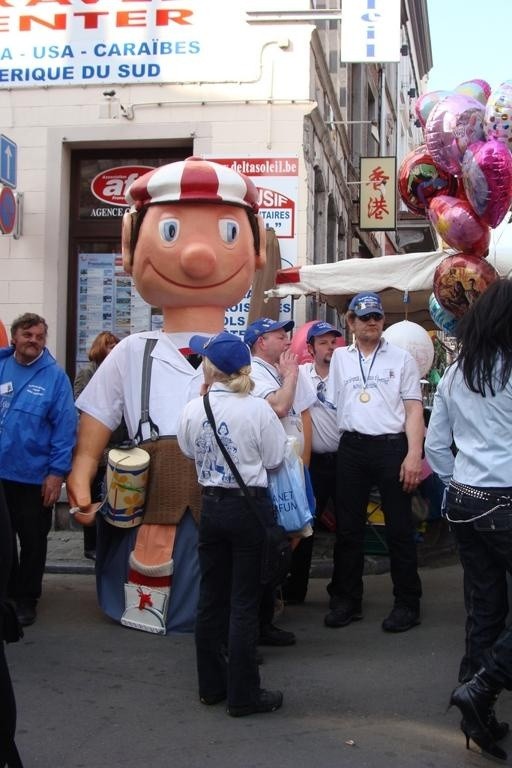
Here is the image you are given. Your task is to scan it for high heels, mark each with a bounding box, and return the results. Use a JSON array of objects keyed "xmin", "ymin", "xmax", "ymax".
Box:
[
  {"xmin": 456, "ymin": 706, "xmax": 512, "ymax": 760},
  {"xmin": 441, "ymin": 668, "xmax": 512, "ymax": 740}
]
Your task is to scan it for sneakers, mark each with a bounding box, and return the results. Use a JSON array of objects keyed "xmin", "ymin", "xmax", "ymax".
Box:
[
  {"xmin": 201, "ymin": 683, "xmax": 287, "ymax": 718},
  {"xmin": 323, "ymin": 605, "xmax": 364, "ymax": 628},
  {"xmin": 379, "ymin": 595, "xmax": 421, "ymax": 633},
  {"xmin": 15, "ymin": 598, "xmax": 38, "ymax": 627},
  {"xmin": 257, "ymin": 620, "xmax": 297, "ymax": 649}
]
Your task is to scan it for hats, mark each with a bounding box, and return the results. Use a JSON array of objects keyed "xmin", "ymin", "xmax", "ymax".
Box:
[
  {"xmin": 348, "ymin": 290, "xmax": 386, "ymax": 316},
  {"xmin": 304, "ymin": 320, "xmax": 344, "ymax": 344},
  {"xmin": 242, "ymin": 316, "xmax": 293, "ymax": 347},
  {"xmin": 187, "ymin": 329, "xmax": 252, "ymax": 376}
]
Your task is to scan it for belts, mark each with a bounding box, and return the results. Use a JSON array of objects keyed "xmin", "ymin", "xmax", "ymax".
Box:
[{"xmin": 344, "ymin": 430, "xmax": 404, "ymax": 442}]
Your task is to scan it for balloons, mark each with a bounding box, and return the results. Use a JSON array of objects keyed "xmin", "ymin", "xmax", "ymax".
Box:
[{"xmin": 397, "ymin": 73, "xmax": 511, "ymax": 337}]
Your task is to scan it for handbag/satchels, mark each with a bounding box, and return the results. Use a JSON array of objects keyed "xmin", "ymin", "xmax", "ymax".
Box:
[
  {"xmin": 259, "ymin": 524, "xmax": 297, "ymax": 586},
  {"xmin": 265, "ymin": 435, "xmax": 316, "ymax": 540}
]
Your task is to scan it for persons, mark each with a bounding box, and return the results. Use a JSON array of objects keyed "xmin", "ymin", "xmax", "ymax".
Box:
[
  {"xmin": 293, "ymin": 319, "xmax": 342, "ymax": 607},
  {"xmin": 421, "ymin": 352, "xmax": 442, "ymax": 389},
  {"xmin": 88, "ymin": 327, "xmax": 118, "ymax": 361},
  {"xmin": 70, "ymin": 155, "xmax": 268, "ymax": 636},
  {"xmin": 177, "ymin": 327, "xmax": 289, "ymax": 716},
  {"xmin": 244, "ymin": 314, "xmax": 307, "ymax": 648},
  {"xmin": 1, "ymin": 620, "xmax": 26, "ymax": 766},
  {"xmin": 0, "ymin": 312, "xmax": 81, "ymax": 625},
  {"xmin": 422, "ymin": 274, "xmax": 512, "ymax": 756},
  {"xmin": 324, "ymin": 291, "xmax": 426, "ymax": 633}
]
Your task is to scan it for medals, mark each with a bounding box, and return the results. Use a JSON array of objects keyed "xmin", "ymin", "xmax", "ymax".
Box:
[{"xmin": 358, "ymin": 392, "xmax": 371, "ymax": 403}]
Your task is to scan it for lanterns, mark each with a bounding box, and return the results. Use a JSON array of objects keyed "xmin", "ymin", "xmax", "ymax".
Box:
[{"xmin": 378, "ymin": 319, "xmax": 433, "ymax": 378}]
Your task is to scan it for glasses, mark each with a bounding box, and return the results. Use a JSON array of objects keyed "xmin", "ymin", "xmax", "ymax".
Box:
[{"xmin": 355, "ymin": 313, "xmax": 384, "ymax": 323}]
